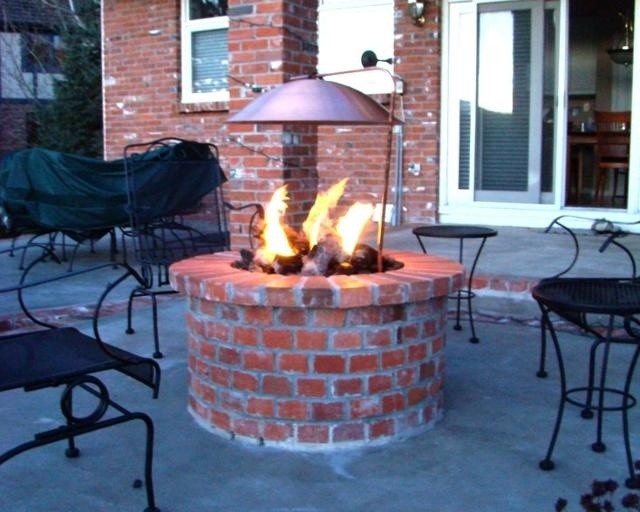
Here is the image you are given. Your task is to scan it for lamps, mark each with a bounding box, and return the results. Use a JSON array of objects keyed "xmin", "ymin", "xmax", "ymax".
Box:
[{"xmin": 603, "ymin": 11, "xmax": 633, "ymax": 66}]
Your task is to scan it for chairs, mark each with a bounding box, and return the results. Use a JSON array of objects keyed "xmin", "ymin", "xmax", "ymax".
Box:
[
  {"xmin": 591, "ymin": 110, "xmax": 632, "ymax": 206},
  {"xmin": 2, "ymin": 243, "xmax": 165, "ymax": 512},
  {"xmin": 121, "ymin": 139, "xmax": 228, "ymax": 360},
  {"xmin": 535, "ymin": 213, "xmax": 640, "ymax": 420},
  {"xmin": 3, "ymin": 135, "xmax": 199, "ymax": 271}
]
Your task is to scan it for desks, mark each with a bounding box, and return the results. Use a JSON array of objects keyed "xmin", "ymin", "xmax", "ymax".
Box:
[
  {"xmin": 564, "ymin": 132, "xmax": 597, "ymax": 203},
  {"xmin": 529, "ymin": 280, "xmax": 640, "ymax": 489},
  {"xmin": 411, "ymin": 226, "xmax": 497, "ymax": 344}
]
